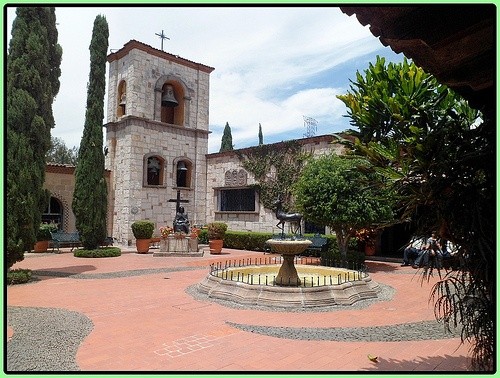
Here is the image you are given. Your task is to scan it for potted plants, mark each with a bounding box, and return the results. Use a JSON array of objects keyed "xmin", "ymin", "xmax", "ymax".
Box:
[
  {"xmin": 34, "ymin": 221, "xmax": 59, "ymax": 253},
  {"xmin": 131, "ymin": 219, "xmax": 155, "ymax": 254},
  {"xmin": 350, "ymin": 227, "xmax": 379, "ymax": 256},
  {"xmin": 207, "ymin": 219, "xmax": 228, "ymax": 255}
]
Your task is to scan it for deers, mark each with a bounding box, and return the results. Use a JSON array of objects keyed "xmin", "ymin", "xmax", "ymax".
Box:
[{"xmin": 270, "ymin": 185, "xmax": 304, "ymax": 240}]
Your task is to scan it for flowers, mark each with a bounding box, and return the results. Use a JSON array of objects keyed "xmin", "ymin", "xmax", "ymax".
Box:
[
  {"xmin": 159, "ymin": 225, "xmax": 174, "ymax": 239},
  {"xmin": 190, "ymin": 227, "xmax": 201, "ymax": 240},
  {"xmin": 173, "ymin": 228, "xmax": 187, "ymax": 239}
]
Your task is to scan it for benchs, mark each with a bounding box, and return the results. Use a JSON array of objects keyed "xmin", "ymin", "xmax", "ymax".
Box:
[
  {"xmin": 50, "ymin": 231, "xmax": 81, "ymax": 254},
  {"xmin": 298, "ymin": 236, "xmax": 327, "ymax": 259}
]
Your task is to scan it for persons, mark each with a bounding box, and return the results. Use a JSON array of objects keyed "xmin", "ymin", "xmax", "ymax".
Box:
[
  {"xmin": 423, "ymin": 230, "xmax": 470, "ymax": 270},
  {"xmin": 400, "ymin": 229, "xmax": 426, "ymax": 269}
]
